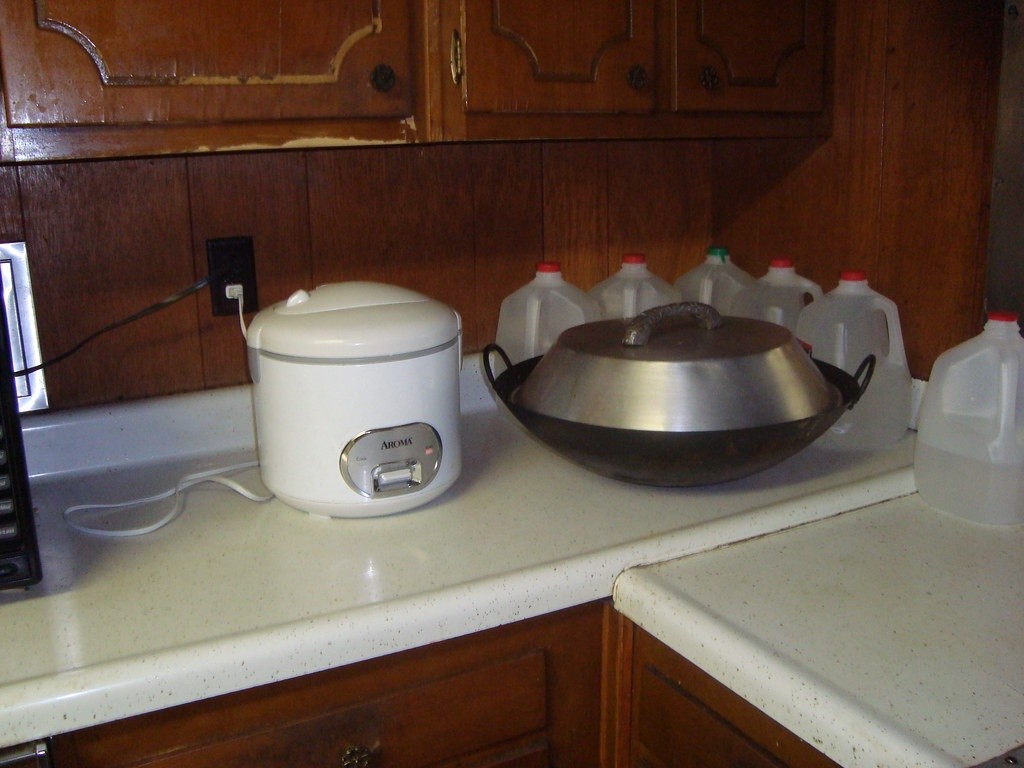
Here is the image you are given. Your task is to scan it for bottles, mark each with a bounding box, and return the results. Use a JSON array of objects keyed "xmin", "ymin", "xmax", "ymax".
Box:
[
  {"xmin": 790, "ymin": 271, "xmax": 912, "ymax": 445},
  {"xmin": 673, "ymin": 245, "xmax": 758, "ymax": 320},
  {"xmin": 493, "ymin": 263, "xmax": 599, "ymax": 376},
  {"xmin": 584, "ymin": 254, "xmax": 681, "ymax": 323},
  {"xmin": 913, "ymin": 311, "xmax": 1024, "ymax": 527},
  {"xmin": 728, "ymin": 258, "xmax": 823, "ymax": 335}
]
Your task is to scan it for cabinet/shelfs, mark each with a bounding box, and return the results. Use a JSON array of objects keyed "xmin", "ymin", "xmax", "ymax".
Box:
[
  {"xmin": 58, "ymin": 595, "xmax": 618, "ymax": 768},
  {"xmin": 0, "ymin": 0, "xmax": 841, "ymax": 163},
  {"xmin": 618, "ymin": 618, "xmax": 841, "ymax": 768}
]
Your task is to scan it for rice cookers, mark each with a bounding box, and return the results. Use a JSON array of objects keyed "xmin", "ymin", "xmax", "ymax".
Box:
[{"xmin": 245, "ymin": 280, "xmax": 465, "ymax": 521}]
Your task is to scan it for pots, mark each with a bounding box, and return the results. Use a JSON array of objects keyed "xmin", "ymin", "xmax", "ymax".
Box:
[{"xmin": 484, "ymin": 302, "xmax": 878, "ymax": 487}]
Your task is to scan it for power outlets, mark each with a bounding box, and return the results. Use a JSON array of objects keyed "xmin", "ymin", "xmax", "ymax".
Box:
[{"xmin": 207, "ymin": 235, "xmax": 258, "ymax": 314}]
